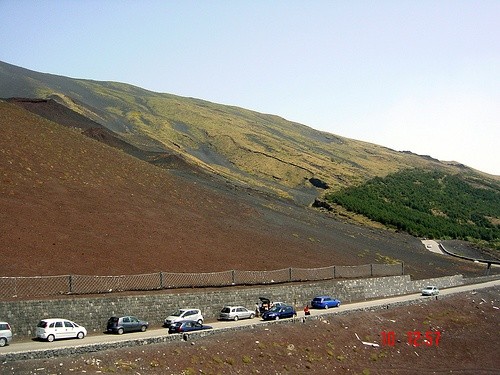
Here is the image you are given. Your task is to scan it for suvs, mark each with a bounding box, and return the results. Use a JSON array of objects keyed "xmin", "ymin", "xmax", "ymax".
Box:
[
  {"xmin": 220, "ymin": 305, "xmax": 256, "ymax": 321},
  {"xmin": 0, "ymin": 322, "xmax": 12, "ymax": 347}
]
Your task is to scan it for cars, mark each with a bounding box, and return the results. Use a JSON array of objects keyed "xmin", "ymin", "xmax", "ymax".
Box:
[
  {"xmin": 421, "ymin": 286, "xmax": 440, "ymax": 296},
  {"xmin": 168, "ymin": 320, "xmax": 213, "ymax": 334},
  {"xmin": 258, "ymin": 296, "xmax": 288, "ymax": 315},
  {"xmin": 164, "ymin": 308, "xmax": 204, "ymax": 326},
  {"xmin": 311, "ymin": 295, "xmax": 342, "ymax": 309},
  {"xmin": 107, "ymin": 315, "xmax": 150, "ymax": 335},
  {"xmin": 36, "ymin": 318, "xmax": 88, "ymax": 342},
  {"xmin": 261, "ymin": 305, "xmax": 297, "ymax": 321}
]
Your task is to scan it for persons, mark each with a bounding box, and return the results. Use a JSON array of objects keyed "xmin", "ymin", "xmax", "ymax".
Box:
[
  {"xmin": 255, "ymin": 302, "xmax": 260, "ymax": 316},
  {"xmin": 304, "ymin": 305, "xmax": 311, "ymax": 315},
  {"xmin": 271, "ymin": 301, "xmax": 274, "ymax": 306}
]
